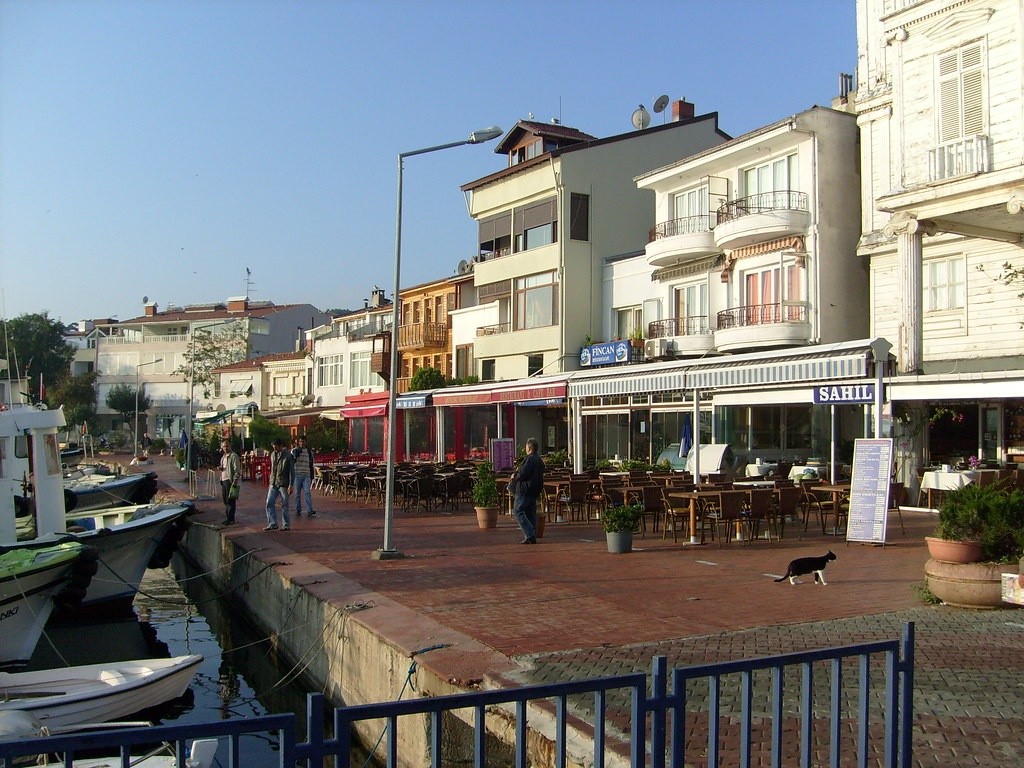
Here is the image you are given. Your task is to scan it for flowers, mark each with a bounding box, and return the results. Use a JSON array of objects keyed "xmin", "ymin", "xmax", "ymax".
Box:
[{"xmin": 891, "ymin": 405, "xmax": 965, "ymax": 483}]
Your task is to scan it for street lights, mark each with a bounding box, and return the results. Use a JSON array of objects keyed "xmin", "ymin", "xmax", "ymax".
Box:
[
  {"xmin": 132, "ymin": 358, "xmax": 163, "ymax": 457},
  {"xmin": 185, "ymin": 318, "xmax": 236, "ymax": 479},
  {"xmin": 378, "ymin": 125, "xmax": 505, "ymax": 554}
]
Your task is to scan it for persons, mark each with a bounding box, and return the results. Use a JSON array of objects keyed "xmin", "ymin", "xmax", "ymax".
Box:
[
  {"xmin": 185, "ymin": 434, "xmax": 200, "ymax": 471},
  {"xmin": 513, "ymin": 438, "xmax": 544, "ymax": 544},
  {"xmin": 291, "ymin": 436, "xmax": 317, "ymax": 516},
  {"xmin": 217, "ymin": 438, "xmax": 241, "ymax": 526},
  {"xmin": 139, "ymin": 433, "xmax": 152, "ymax": 457},
  {"xmin": 263, "ymin": 438, "xmax": 296, "ymax": 530}
]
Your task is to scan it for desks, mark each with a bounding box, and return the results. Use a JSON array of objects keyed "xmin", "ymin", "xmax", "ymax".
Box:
[
  {"xmin": 319, "ymin": 469, "xmax": 332, "ymax": 496},
  {"xmin": 600, "ymin": 471, "xmax": 653, "ymax": 487},
  {"xmin": 732, "ymin": 481, "xmax": 775, "ymax": 488},
  {"xmin": 339, "ymin": 472, "xmax": 357, "ymax": 503},
  {"xmin": 433, "ymin": 472, "xmax": 453, "ymax": 514},
  {"xmin": 668, "ymin": 489, "xmax": 737, "ymax": 544},
  {"xmin": 975, "ymin": 468, "xmax": 1004, "ymax": 487},
  {"xmin": 607, "ymin": 486, "xmax": 643, "ymax": 508},
  {"xmin": 811, "ymin": 484, "xmax": 851, "ymax": 535},
  {"xmin": 920, "ymin": 472, "xmax": 972, "ymax": 509},
  {"xmin": 455, "ymin": 467, "xmax": 475, "ymax": 504},
  {"xmin": 495, "ymin": 477, "xmax": 514, "ymax": 517},
  {"xmin": 365, "ymin": 475, "xmax": 385, "ymax": 505},
  {"xmin": 543, "ymin": 477, "xmax": 599, "ymax": 523}
]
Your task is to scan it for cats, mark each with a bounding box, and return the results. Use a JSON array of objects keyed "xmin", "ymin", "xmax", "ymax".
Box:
[{"xmin": 774, "ymin": 550, "xmax": 836, "ymax": 586}]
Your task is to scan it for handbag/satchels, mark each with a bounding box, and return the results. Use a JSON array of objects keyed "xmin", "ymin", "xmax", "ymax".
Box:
[
  {"xmin": 507, "ymin": 478, "xmax": 516, "ymax": 496},
  {"xmin": 227, "ymin": 485, "xmax": 240, "ymax": 499},
  {"xmin": 219, "ymin": 476, "xmax": 222, "ymax": 484}
]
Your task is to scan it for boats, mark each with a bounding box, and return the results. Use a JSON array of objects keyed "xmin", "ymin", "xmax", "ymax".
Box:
[{"xmin": 0, "ymin": 375, "xmax": 221, "ymax": 768}]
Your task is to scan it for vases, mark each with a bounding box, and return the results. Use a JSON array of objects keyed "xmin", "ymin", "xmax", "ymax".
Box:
[
  {"xmin": 614, "ymin": 338, "xmax": 620, "ymax": 342},
  {"xmin": 888, "ymin": 483, "xmax": 903, "ymax": 508}
]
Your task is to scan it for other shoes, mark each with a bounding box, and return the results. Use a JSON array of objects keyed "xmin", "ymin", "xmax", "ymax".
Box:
[
  {"xmin": 222, "ymin": 519, "xmax": 235, "ymax": 525},
  {"xmin": 296, "ymin": 510, "xmax": 299, "ymax": 515},
  {"xmin": 306, "ymin": 509, "xmax": 316, "ymax": 515},
  {"xmin": 278, "ymin": 526, "xmax": 289, "ymax": 531},
  {"xmin": 520, "ymin": 538, "xmax": 536, "ymax": 544},
  {"xmin": 263, "ymin": 525, "xmax": 276, "ymax": 530}
]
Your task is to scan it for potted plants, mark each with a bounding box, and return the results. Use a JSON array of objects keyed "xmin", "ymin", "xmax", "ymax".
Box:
[
  {"xmin": 515, "ymin": 447, "xmax": 545, "ymax": 538},
  {"xmin": 629, "ymin": 329, "xmax": 644, "ymax": 348},
  {"xmin": 600, "ymin": 503, "xmax": 644, "ymax": 553},
  {"xmin": 472, "ymin": 462, "xmax": 499, "ymax": 529},
  {"xmin": 925, "ymin": 475, "xmax": 1024, "ymax": 609}
]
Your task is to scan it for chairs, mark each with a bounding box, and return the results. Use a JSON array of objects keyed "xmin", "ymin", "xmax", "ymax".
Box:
[{"xmin": 247, "ymin": 451, "xmax": 904, "ymax": 549}]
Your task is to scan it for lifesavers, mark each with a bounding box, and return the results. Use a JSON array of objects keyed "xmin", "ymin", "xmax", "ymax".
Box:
[
  {"xmin": 13, "ymin": 495, "xmax": 29, "ymax": 517},
  {"xmin": 64, "ymin": 489, "xmax": 77, "ymax": 513}
]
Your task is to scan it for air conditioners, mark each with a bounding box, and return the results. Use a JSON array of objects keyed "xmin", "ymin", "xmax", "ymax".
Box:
[{"xmin": 644, "ymin": 339, "xmax": 667, "ymax": 358}]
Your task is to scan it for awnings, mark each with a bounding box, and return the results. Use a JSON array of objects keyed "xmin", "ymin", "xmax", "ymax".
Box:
[{"xmin": 193, "ymin": 348, "xmax": 871, "ymax": 425}]
